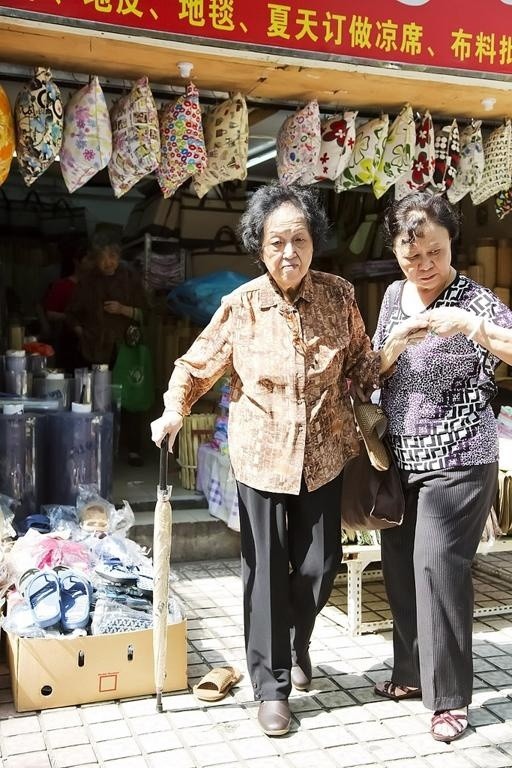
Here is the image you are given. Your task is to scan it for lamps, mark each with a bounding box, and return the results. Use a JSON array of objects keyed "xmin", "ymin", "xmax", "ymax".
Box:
[{"xmin": 177, "ymin": 61, "xmax": 194, "ymax": 77}]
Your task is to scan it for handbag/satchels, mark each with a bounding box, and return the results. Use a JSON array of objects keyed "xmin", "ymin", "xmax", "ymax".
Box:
[
  {"xmin": 110, "ymin": 342, "xmax": 156, "ymax": 412},
  {"xmin": 340, "ymin": 442, "xmax": 407, "ymax": 530},
  {"xmin": 191, "ymin": 226, "xmax": 266, "ymax": 280},
  {"xmin": 121, "ymin": 190, "xmax": 181, "ymax": 241},
  {"xmin": 180, "ymin": 185, "xmax": 251, "ymax": 244},
  {"xmin": 126, "ymin": 324, "xmax": 141, "ymax": 346},
  {"xmin": 0, "ymin": 187, "xmax": 87, "ymax": 237},
  {"xmin": 316, "ymin": 200, "xmax": 385, "ymax": 260}
]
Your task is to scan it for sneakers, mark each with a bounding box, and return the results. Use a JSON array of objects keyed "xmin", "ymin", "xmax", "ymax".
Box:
[{"xmin": 127, "ymin": 452, "xmax": 144, "ymax": 467}]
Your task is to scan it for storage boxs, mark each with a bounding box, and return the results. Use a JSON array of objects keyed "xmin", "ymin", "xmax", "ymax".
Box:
[{"xmin": 4, "ymin": 589, "xmax": 188, "ymax": 713}]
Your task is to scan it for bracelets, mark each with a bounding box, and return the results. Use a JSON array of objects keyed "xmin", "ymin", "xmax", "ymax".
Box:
[{"xmin": 465, "ymin": 318, "xmax": 485, "ymax": 342}]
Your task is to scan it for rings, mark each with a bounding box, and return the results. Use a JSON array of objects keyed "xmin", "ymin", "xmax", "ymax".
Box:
[{"xmin": 430, "ymin": 331, "xmax": 437, "ymax": 336}]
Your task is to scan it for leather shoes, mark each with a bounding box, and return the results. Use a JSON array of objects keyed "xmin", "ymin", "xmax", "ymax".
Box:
[
  {"xmin": 258, "ymin": 700, "xmax": 291, "ymax": 736},
  {"xmin": 290, "ymin": 653, "xmax": 313, "ymax": 689}
]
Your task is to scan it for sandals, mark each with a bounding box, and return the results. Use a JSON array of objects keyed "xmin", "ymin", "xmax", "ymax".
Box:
[
  {"xmin": 374, "ymin": 678, "xmax": 421, "ymax": 699},
  {"xmin": 430, "ymin": 706, "xmax": 469, "ymax": 740}
]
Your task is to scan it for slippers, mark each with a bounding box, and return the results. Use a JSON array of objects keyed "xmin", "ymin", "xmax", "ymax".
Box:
[
  {"xmin": 352, "ymin": 397, "xmax": 389, "ymax": 471},
  {"xmin": 192, "ymin": 666, "xmax": 238, "ymax": 701},
  {"xmin": 5, "ymin": 501, "xmax": 154, "ymax": 631}
]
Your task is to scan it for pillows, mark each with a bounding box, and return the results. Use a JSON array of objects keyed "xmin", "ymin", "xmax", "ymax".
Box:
[
  {"xmin": 447, "ymin": 118, "xmax": 489, "ymax": 207},
  {"xmin": 192, "ymin": 91, "xmax": 251, "ymax": 202},
  {"xmin": 333, "ymin": 110, "xmax": 392, "ymax": 194},
  {"xmin": 156, "ymin": 80, "xmax": 209, "ymax": 200},
  {"xmin": 393, "ymin": 109, "xmax": 440, "ymax": 203},
  {"xmin": 106, "ymin": 75, "xmax": 162, "ymax": 200},
  {"xmin": 494, "ymin": 188, "xmax": 512, "ymax": 220},
  {"xmin": 16, "ymin": 66, "xmax": 65, "ymax": 187},
  {"xmin": 60, "ymin": 75, "xmax": 113, "ymax": 195},
  {"xmin": 294, "ymin": 109, "xmax": 363, "ymax": 188},
  {"xmin": 0, "ymin": 86, "xmax": 15, "ymax": 187},
  {"xmin": 371, "ymin": 101, "xmax": 419, "ymax": 200},
  {"xmin": 469, "ymin": 119, "xmax": 512, "ymax": 206},
  {"xmin": 421, "ymin": 118, "xmax": 461, "ymax": 202},
  {"xmin": 274, "ymin": 95, "xmax": 326, "ymax": 187}
]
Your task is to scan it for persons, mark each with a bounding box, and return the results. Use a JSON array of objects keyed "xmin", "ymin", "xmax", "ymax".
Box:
[
  {"xmin": 43, "ymin": 238, "xmax": 96, "ymax": 370},
  {"xmin": 145, "ymin": 179, "xmax": 384, "ymax": 740},
  {"xmin": 367, "ymin": 187, "xmax": 512, "ymax": 746},
  {"xmin": 63, "ymin": 228, "xmax": 152, "ymax": 470}
]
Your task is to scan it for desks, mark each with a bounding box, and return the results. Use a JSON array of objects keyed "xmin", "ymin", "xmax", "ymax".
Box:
[
  {"xmin": 1, "ymin": 391, "xmax": 60, "ymax": 494},
  {"xmin": 318, "ymin": 538, "xmax": 511, "ymax": 634},
  {"xmin": 194, "ymin": 443, "xmax": 241, "ymax": 532}
]
